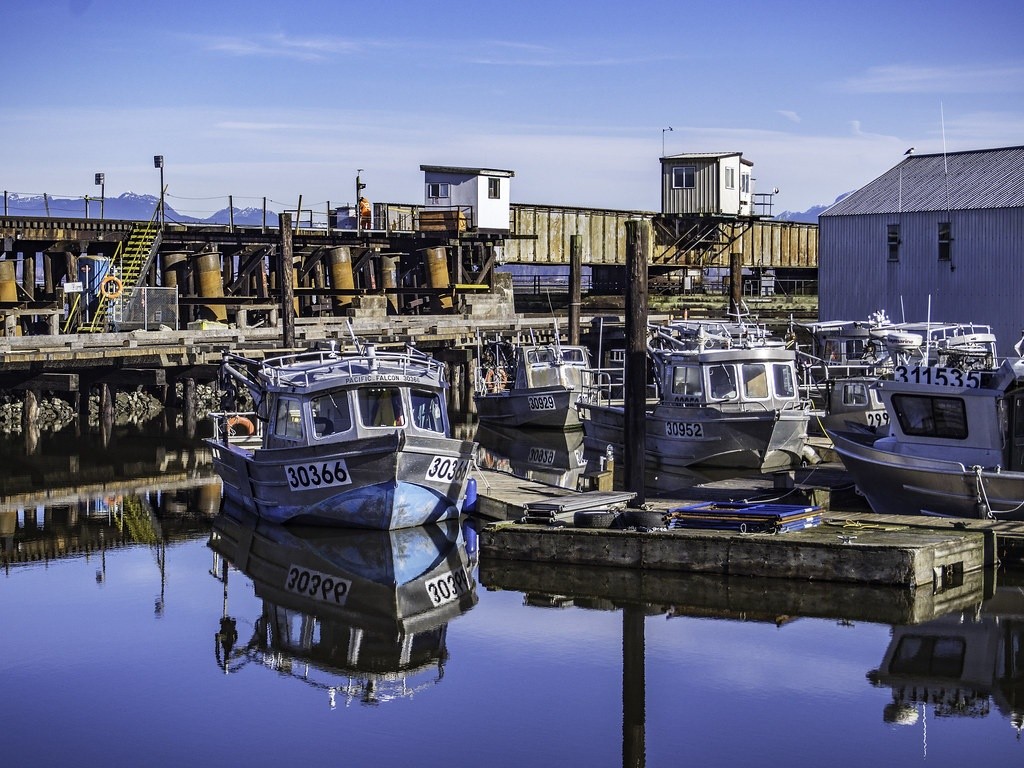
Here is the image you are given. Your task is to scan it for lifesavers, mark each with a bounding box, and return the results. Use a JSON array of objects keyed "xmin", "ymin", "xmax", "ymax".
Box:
[
  {"xmin": 101, "ymin": 276, "xmax": 122, "ymax": 299},
  {"xmin": 486, "ymin": 369, "xmax": 507, "ymax": 392},
  {"xmin": 227, "ymin": 416, "xmax": 255, "ymax": 436}
]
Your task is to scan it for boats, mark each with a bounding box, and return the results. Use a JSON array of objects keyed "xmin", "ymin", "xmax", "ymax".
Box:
[
  {"xmin": 199, "ymin": 320, "xmax": 481, "ymax": 534},
  {"xmin": 473, "ymin": 292, "xmax": 1024, "ymax": 523},
  {"xmin": 206, "ymin": 497, "xmax": 481, "ymax": 713}
]
